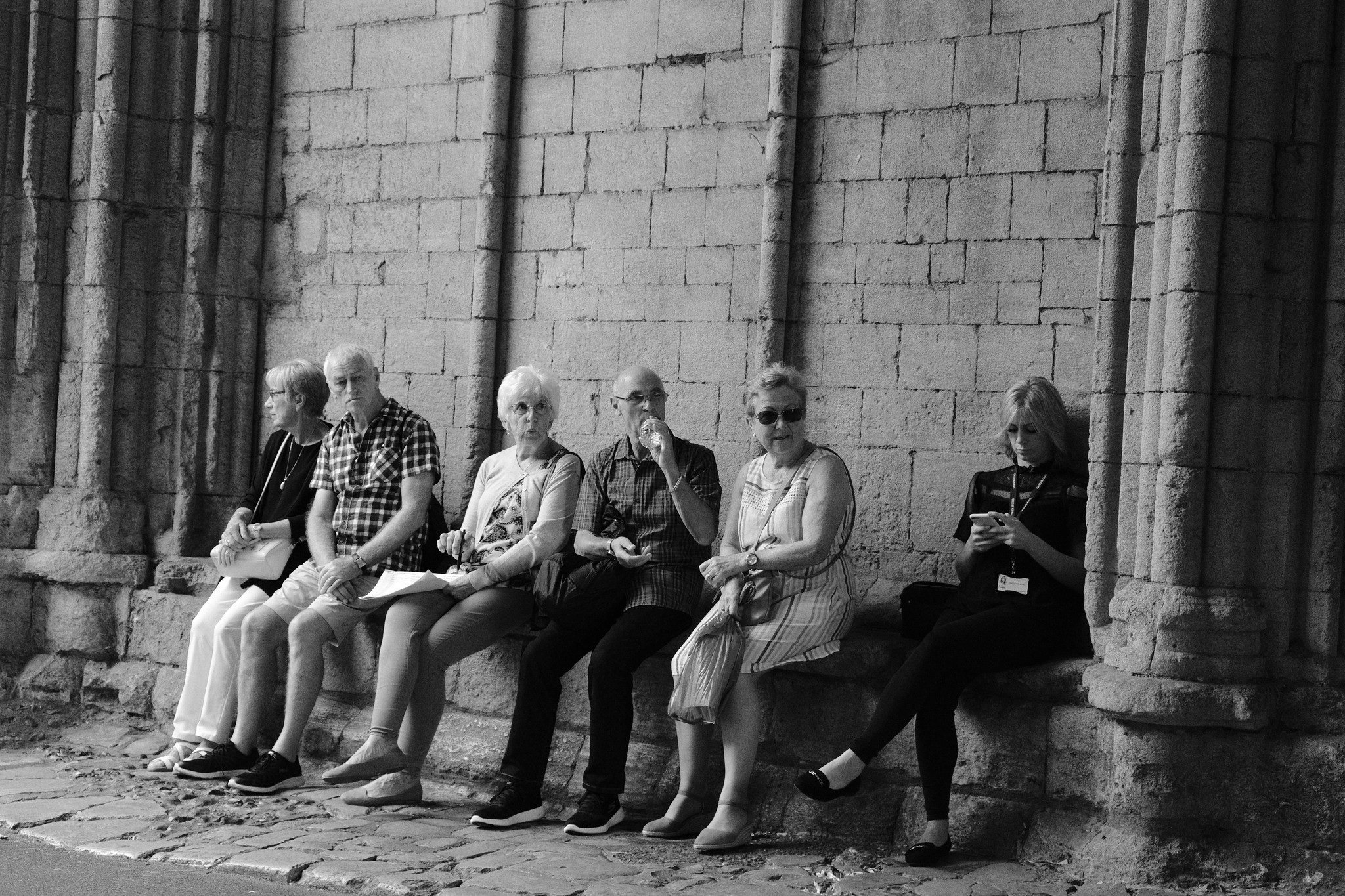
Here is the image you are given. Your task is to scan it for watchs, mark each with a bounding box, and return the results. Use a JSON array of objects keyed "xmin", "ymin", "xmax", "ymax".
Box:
[
  {"xmin": 607, "ymin": 538, "xmax": 616, "ymax": 559},
  {"xmin": 252, "ymin": 523, "xmax": 262, "ymax": 539},
  {"xmin": 352, "ymin": 554, "xmax": 367, "ymax": 573},
  {"xmin": 745, "ymin": 550, "xmax": 758, "ymax": 571}
]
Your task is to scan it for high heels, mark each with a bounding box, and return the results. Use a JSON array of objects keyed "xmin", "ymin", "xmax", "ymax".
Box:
[
  {"xmin": 694, "ymin": 801, "xmax": 753, "ymax": 849},
  {"xmin": 642, "ymin": 790, "xmax": 717, "ymax": 836}
]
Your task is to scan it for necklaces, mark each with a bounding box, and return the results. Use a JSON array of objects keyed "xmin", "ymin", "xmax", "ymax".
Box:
[
  {"xmin": 280, "ymin": 418, "xmax": 320, "ymax": 490},
  {"xmin": 524, "ymin": 436, "xmax": 549, "ymax": 471}
]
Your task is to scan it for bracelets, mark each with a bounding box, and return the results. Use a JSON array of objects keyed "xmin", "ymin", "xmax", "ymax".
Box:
[{"xmin": 668, "ymin": 471, "xmax": 682, "ymax": 492}]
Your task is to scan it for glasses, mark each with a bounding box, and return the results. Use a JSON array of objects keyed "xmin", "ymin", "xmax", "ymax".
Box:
[
  {"xmin": 616, "ymin": 391, "xmax": 669, "ymax": 408},
  {"xmin": 507, "ymin": 401, "xmax": 553, "ymax": 415},
  {"xmin": 270, "ymin": 391, "xmax": 285, "ymax": 400},
  {"xmin": 752, "ymin": 408, "xmax": 805, "ymax": 425}
]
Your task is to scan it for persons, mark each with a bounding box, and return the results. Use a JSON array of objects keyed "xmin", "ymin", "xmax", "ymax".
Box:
[
  {"xmin": 146, "ymin": 361, "xmax": 336, "ymax": 772},
  {"xmin": 795, "ymin": 376, "xmax": 1089, "ymax": 866},
  {"xmin": 467, "ymin": 364, "xmax": 721, "ymax": 835},
  {"xmin": 322, "ymin": 367, "xmax": 580, "ymax": 806},
  {"xmin": 641, "ymin": 362, "xmax": 857, "ymax": 852},
  {"xmin": 171, "ymin": 345, "xmax": 440, "ymax": 791},
  {"xmin": 1000, "ymin": 576, "xmax": 1007, "ymax": 585}
]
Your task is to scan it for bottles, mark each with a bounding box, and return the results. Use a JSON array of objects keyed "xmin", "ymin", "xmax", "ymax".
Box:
[{"xmin": 639, "ymin": 416, "xmax": 662, "ymax": 448}]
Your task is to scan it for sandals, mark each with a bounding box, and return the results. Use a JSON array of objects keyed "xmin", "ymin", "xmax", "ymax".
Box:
[
  {"xmin": 172, "ymin": 747, "xmax": 214, "ymax": 776},
  {"xmin": 147, "ymin": 741, "xmax": 200, "ymax": 771}
]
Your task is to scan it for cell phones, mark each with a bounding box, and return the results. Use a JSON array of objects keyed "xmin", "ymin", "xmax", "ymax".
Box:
[{"xmin": 970, "ymin": 513, "xmax": 1000, "ymax": 529}]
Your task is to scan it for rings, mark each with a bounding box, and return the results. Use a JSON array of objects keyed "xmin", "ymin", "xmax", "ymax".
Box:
[
  {"xmin": 225, "ymin": 539, "xmax": 229, "ymax": 542},
  {"xmin": 225, "ymin": 550, "xmax": 229, "ymax": 556},
  {"xmin": 706, "ymin": 572, "xmax": 713, "ymax": 581},
  {"xmin": 667, "ymin": 430, "xmax": 670, "ymax": 434}
]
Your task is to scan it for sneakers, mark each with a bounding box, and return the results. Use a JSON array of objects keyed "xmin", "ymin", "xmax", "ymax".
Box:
[
  {"xmin": 470, "ymin": 782, "xmax": 546, "ymax": 828},
  {"xmin": 174, "ymin": 741, "xmax": 260, "ymax": 779},
  {"xmin": 228, "ymin": 750, "xmax": 305, "ymax": 793},
  {"xmin": 564, "ymin": 789, "xmax": 625, "ymax": 834}
]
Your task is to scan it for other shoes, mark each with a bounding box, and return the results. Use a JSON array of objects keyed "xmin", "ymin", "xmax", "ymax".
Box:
[
  {"xmin": 793, "ymin": 769, "xmax": 861, "ymax": 803},
  {"xmin": 905, "ymin": 834, "xmax": 952, "ymax": 864},
  {"xmin": 322, "ymin": 747, "xmax": 407, "ymax": 783},
  {"xmin": 342, "ymin": 771, "xmax": 423, "ymax": 805}
]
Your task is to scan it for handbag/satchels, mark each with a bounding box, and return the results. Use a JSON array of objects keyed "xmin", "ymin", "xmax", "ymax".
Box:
[
  {"xmin": 712, "ymin": 569, "xmax": 775, "ymax": 627},
  {"xmin": 533, "ymin": 504, "xmax": 637, "ymax": 631},
  {"xmin": 899, "ymin": 580, "xmax": 960, "ymax": 638},
  {"xmin": 667, "ymin": 580, "xmax": 757, "ymax": 726},
  {"xmin": 210, "ymin": 536, "xmax": 297, "ymax": 580}
]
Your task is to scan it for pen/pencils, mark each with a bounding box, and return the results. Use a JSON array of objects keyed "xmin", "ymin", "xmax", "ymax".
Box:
[{"xmin": 456, "ymin": 528, "xmax": 466, "ymax": 573}]
